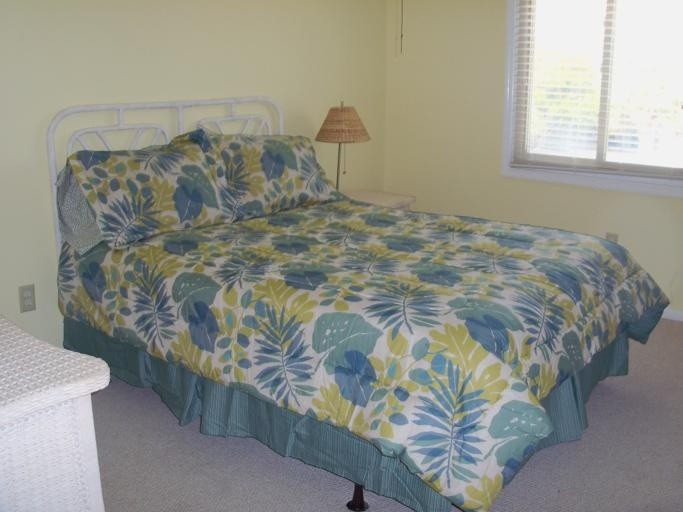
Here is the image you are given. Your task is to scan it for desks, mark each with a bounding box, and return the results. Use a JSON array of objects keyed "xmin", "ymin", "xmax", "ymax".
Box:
[{"xmin": 0, "ymin": 313, "xmax": 112, "ymax": 512}]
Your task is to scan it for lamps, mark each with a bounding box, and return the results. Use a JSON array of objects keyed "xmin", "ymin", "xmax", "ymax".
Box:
[{"xmin": 315, "ymin": 100, "xmax": 371, "ymax": 188}]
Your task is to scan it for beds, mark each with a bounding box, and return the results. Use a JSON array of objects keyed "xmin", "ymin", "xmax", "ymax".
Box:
[{"xmin": 45, "ymin": 96, "xmax": 671, "ymax": 512}]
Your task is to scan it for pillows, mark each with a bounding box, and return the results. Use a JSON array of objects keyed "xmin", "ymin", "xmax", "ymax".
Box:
[
  {"xmin": 67, "ymin": 130, "xmax": 229, "ymax": 247},
  {"xmin": 206, "ymin": 132, "xmax": 345, "ymax": 224}
]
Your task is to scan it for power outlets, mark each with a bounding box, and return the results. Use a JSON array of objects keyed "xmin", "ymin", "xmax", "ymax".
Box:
[{"xmin": 18, "ymin": 283, "xmax": 36, "ymax": 313}]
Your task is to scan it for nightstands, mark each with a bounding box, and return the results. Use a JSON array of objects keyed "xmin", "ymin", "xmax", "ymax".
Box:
[{"xmin": 345, "ymin": 189, "xmax": 416, "ymax": 211}]
